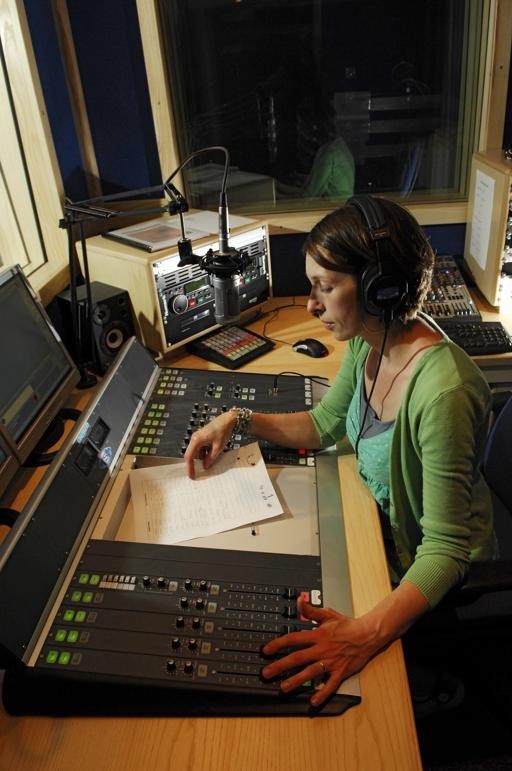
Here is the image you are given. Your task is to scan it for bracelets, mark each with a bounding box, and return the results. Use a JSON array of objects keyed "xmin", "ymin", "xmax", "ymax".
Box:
[{"xmin": 229, "ymin": 406, "xmax": 252, "ymax": 438}]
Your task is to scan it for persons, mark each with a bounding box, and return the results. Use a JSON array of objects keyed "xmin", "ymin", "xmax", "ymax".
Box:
[
  {"xmin": 181, "ymin": 193, "xmax": 499, "ymax": 708},
  {"xmin": 274, "ymin": 102, "xmax": 356, "ymax": 200}
]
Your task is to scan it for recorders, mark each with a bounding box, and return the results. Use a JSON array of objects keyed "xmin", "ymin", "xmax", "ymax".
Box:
[{"xmin": 75, "ymin": 203, "xmax": 274, "ymax": 354}]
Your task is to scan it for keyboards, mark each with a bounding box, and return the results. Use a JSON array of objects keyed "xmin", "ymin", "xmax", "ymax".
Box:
[{"xmin": 436, "ymin": 320, "xmax": 512, "ymax": 355}]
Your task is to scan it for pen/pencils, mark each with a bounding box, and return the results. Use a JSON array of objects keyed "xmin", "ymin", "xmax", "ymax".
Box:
[{"xmin": 252, "ymin": 522, "xmax": 256, "ymax": 536}]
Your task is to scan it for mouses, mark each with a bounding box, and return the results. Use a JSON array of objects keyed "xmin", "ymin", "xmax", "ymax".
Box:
[{"xmin": 294, "ymin": 339, "xmax": 329, "ymax": 357}]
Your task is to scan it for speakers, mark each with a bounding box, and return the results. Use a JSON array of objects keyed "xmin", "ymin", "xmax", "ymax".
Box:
[{"xmin": 56, "ymin": 280, "xmax": 142, "ymax": 377}]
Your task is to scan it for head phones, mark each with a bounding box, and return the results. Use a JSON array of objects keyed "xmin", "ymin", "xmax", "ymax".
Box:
[{"xmin": 346, "ymin": 194, "xmax": 412, "ymax": 321}]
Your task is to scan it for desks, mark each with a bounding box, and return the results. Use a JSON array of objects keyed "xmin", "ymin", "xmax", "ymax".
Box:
[{"xmin": 0, "ymin": 267, "xmax": 512, "ymax": 771}]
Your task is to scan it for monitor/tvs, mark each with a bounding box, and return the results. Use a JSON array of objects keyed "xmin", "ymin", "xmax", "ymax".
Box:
[
  {"xmin": 0, "ymin": 434, "xmax": 20, "ymax": 503},
  {"xmin": 0, "ymin": 263, "xmax": 82, "ymax": 465}
]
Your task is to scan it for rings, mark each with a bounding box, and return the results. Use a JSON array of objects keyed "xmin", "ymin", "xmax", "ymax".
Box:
[{"xmin": 318, "ymin": 660, "xmax": 327, "ymax": 675}]
